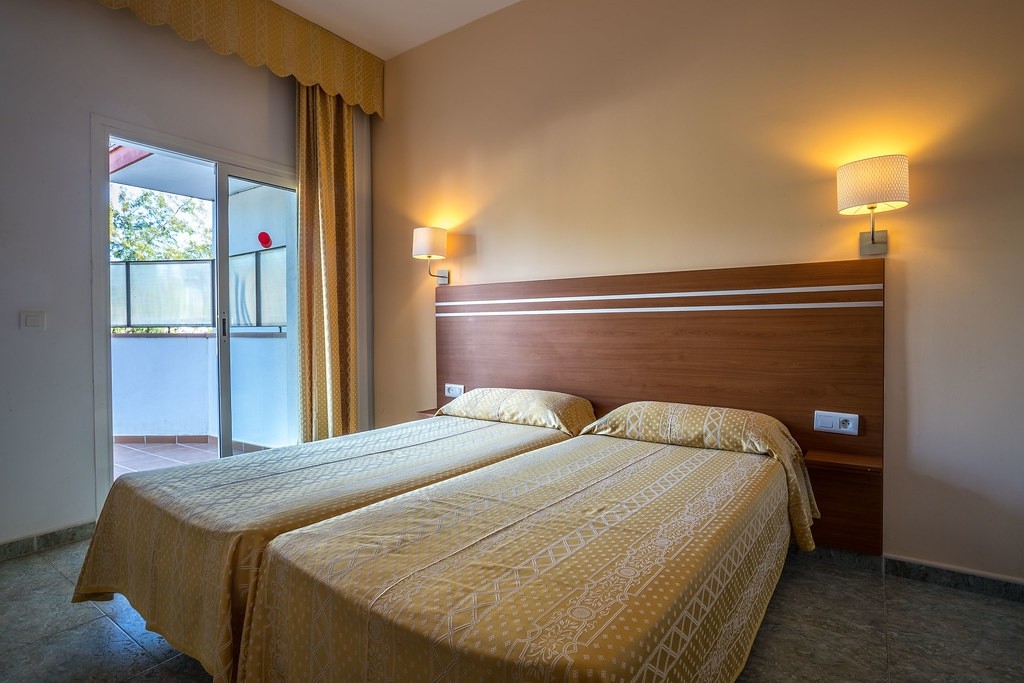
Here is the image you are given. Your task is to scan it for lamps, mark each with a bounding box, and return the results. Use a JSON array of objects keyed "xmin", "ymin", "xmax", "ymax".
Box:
[
  {"xmin": 413, "ymin": 227, "xmax": 450, "ymax": 285},
  {"xmin": 837, "ymin": 153, "xmax": 910, "ymax": 258}
]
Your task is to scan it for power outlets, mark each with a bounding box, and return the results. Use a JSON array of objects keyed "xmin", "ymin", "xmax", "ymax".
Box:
[
  {"xmin": 814, "ymin": 410, "xmax": 858, "ymax": 436},
  {"xmin": 444, "ymin": 383, "xmax": 465, "ymax": 398}
]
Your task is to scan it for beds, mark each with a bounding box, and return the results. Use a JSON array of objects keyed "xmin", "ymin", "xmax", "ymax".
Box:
[{"xmin": 71, "ymin": 256, "xmax": 885, "ymax": 683}]
